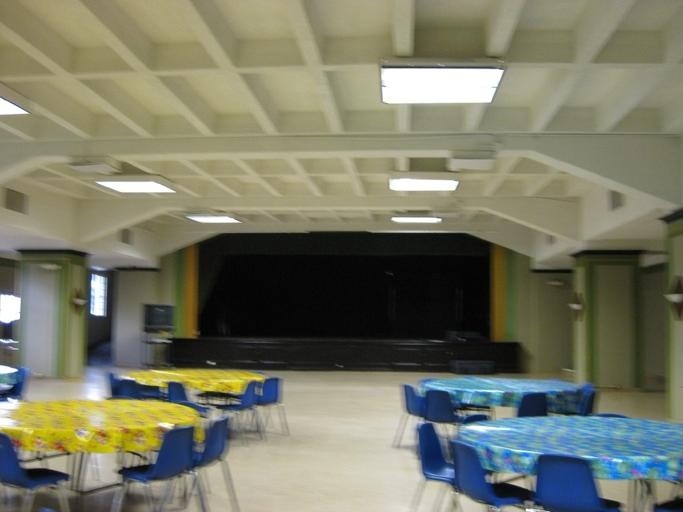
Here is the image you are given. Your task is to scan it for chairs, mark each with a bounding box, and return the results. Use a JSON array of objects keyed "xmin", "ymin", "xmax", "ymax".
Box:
[
  {"xmin": 0, "ymin": 368, "xmax": 30, "ymax": 402},
  {"xmin": 395, "ymin": 377, "xmax": 630, "ymax": 512},
  {"xmin": 169, "ymin": 416, "xmax": 238, "ymax": 511},
  {"xmin": 0, "ymin": 433, "xmax": 74, "ymax": 511},
  {"xmin": 110, "ymin": 424, "xmax": 195, "ymax": 512},
  {"xmin": 102, "ymin": 369, "xmax": 288, "ymax": 449}
]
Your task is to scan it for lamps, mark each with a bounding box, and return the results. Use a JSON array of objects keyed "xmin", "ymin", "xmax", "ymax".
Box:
[
  {"xmin": 378, "ymin": 55, "xmax": 506, "ymax": 104},
  {"xmin": 388, "ymin": 171, "xmax": 461, "ymax": 193},
  {"xmin": 0, "ymin": 82, "xmax": 32, "ymax": 117},
  {"xmin": 96, "ymin": 174, "xmax": 177, "ymax": 194}
]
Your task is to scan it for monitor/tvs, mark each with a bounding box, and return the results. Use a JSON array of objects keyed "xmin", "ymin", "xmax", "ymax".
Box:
[{"xmin": 143, "ymin": 304, "xmax": 176, "ymax": 332}]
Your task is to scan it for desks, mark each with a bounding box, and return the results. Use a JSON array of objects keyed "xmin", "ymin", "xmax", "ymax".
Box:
[
  {"xmin": 455, "ymin": 414, "xmax": 682, "ymax": 512},
  {"xmin": 1, "ymin": 398, "xmax": 208, "ymax": 509}
]
